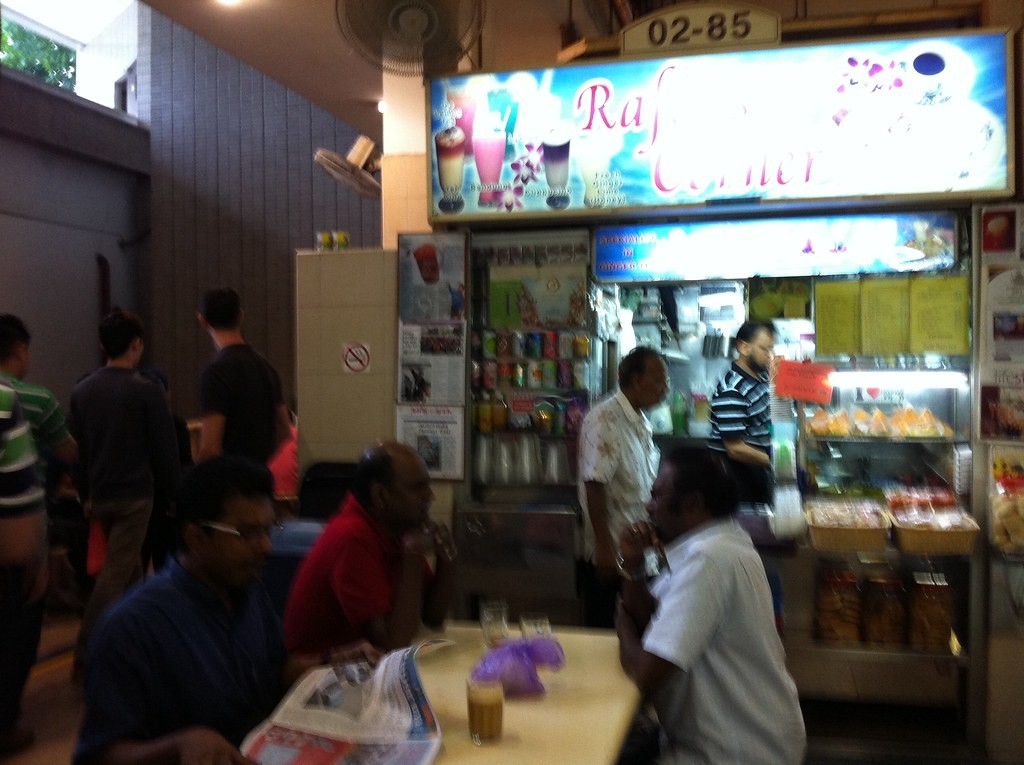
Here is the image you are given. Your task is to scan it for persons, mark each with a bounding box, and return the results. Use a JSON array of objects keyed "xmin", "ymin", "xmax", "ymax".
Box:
[
  {"xmin": 61, "ymin": 311, "xmax": 178, "ymax": 678},
  {"xmin": 284, "ymin": 442, "xmax": 453, "ymax": 655},
  {"xmin": 707, "ymin": 318, "xmax": 783, "ymax": 639},
  {"xmin": 264, "ymin": 384, "xmax": 300, "ymax": 501},
  {"xmin": 195, "ymin": 290, "xmax": 292, "ymax": 536},
  {"xmin": 613, "ymin": 441, "xmax": 806, "ymax": 765},
  {"xmin": 578, "ymin": 346, "xmax": 669, "ymax": 627},
  {"xmin": 0, "ymin": 311, "xmax": 61, "ymax": 758},
  {"xmin": 70, "ymin": 455, "xmax": 379, "ymax": 765}
]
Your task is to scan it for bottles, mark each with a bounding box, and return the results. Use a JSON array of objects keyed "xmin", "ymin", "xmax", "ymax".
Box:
[{"xmin": 474, "ymin": 388, "xmax": 506, "ymax": 434}]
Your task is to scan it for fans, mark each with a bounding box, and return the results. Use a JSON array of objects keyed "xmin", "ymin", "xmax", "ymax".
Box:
[
  {"xmin": 336, "ymin": 0, "xmax": 485, "ymax": 80},
  {"xmin": 314, "ymin": 135, "xmax": 382, "ymax": 199}
]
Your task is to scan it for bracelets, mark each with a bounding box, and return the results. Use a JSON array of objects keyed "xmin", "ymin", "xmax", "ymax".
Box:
[{"xmin": 322, "ymin": 651, "xmax": 329, "ymax": 665}]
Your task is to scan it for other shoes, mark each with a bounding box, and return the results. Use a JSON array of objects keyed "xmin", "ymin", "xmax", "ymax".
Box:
[{"xmin": 0, "ymin": 727, "xmax": 36, "ymax": 758}]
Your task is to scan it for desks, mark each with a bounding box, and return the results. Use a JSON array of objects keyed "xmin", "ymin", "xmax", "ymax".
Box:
[{"xmin": 414, "ymin": 628, "xmax": 645, "ymax": 765}]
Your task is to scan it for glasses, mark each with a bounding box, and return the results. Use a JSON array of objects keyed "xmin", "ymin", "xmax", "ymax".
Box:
[{"xmin": 200, "ymin": 512, "xmax": 288, "ymax": 544}]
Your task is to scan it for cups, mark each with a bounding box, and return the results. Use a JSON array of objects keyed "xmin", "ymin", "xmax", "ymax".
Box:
[
  {"xmin": 466, "ymin": 675, "xmax": 503, "ymax": 747},
  {"xmin": 518, "ymin": 612, "xmax": 552, "ymax": 640},
  {"xmin": 471, "ymin": 131, "xmax": 507, "ymax": 210},
  {"xmin": 579, "ymin": 134, "xmax": 611, "ymax": 208},
  {"xmin": 475, "ymin": 431, "xmax": 571, "ymax": 488},
  {"xmin": 541, "ymin": 132, "xmax": 573, "ymax": 210},
  {"xmin": 434, "ymin": 130, "xmax": 467, "ymax": 215},
  {"xmin": 480, "ymin": 601, "xmax": 510, "ymax": 653}
]
